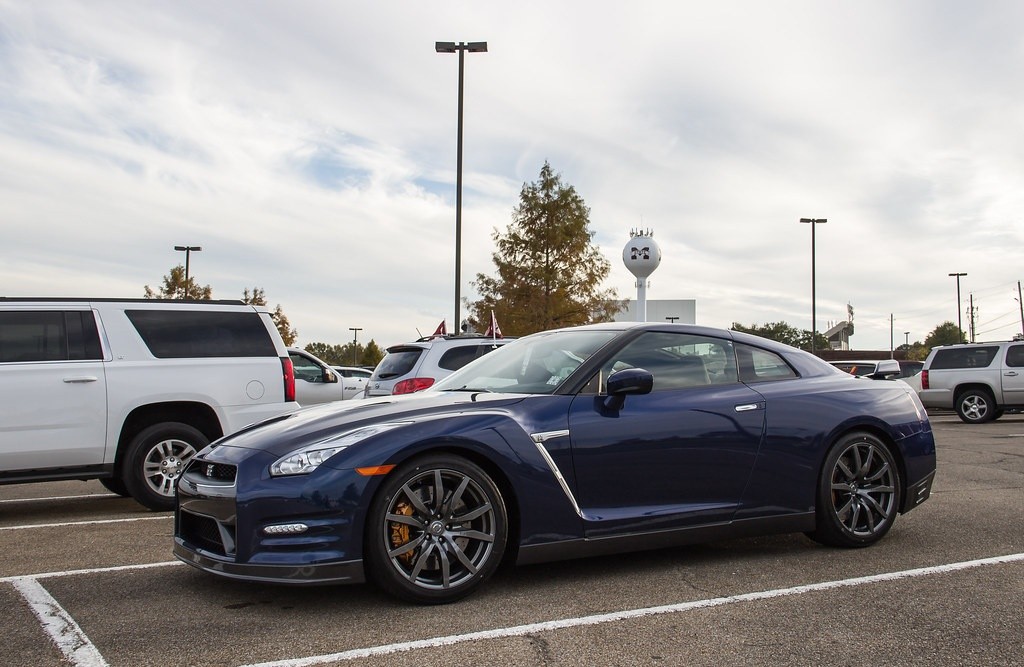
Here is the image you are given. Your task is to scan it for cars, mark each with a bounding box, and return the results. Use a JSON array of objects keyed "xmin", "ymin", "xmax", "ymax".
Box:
[
  {"xmin": 843, "ymin": 360, "xmax": 926, "ymax": 409},
  {"xmin": 293, "ymin": 365, "xmax": 375, "ymax": 378},
  {"xmin": 173, "ymin": 322, "xmax": 937, "ymax": 605}
]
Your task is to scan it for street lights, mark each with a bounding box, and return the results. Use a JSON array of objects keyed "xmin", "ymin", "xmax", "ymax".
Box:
[
  {"xmin": 799, "ymin": 218, "xmax": 828, "ymax": 354},
  {"xmin": 904, "ymin": 331, "xmax": 909, "ymax": 360},
  {"xmin": 949, "ymin": 273, "xmax": 968, "ymax": 343},
  {"xmin": 666, "ymin": 317, "xmax": 679, "ymax": 322},
  {"xmin": 174, "ymin": 246, "xmax": 202, "ymax": 296},
  {"xmin": 435, "ymin": 41, "xmax": 489, "ymax": 332},
  {"xmin": 348, "ymin": 328, "xmax": 363, "ymax": 365}
]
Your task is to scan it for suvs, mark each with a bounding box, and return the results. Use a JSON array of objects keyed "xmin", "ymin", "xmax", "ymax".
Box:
[
  {"xmin": 0, "ymin": 297, "xmax": 301, "ymax": 511},
  {"xmin": 285, "ymin": 346, "xmax": 370, "ymax": 406},
  {"xmin": 363, "ymin": 333, "xmax": 556, "ymax": 402},
  {"xmin": 919, "ymin": 339, "xmax": 1024, "ymax": 423}
]
